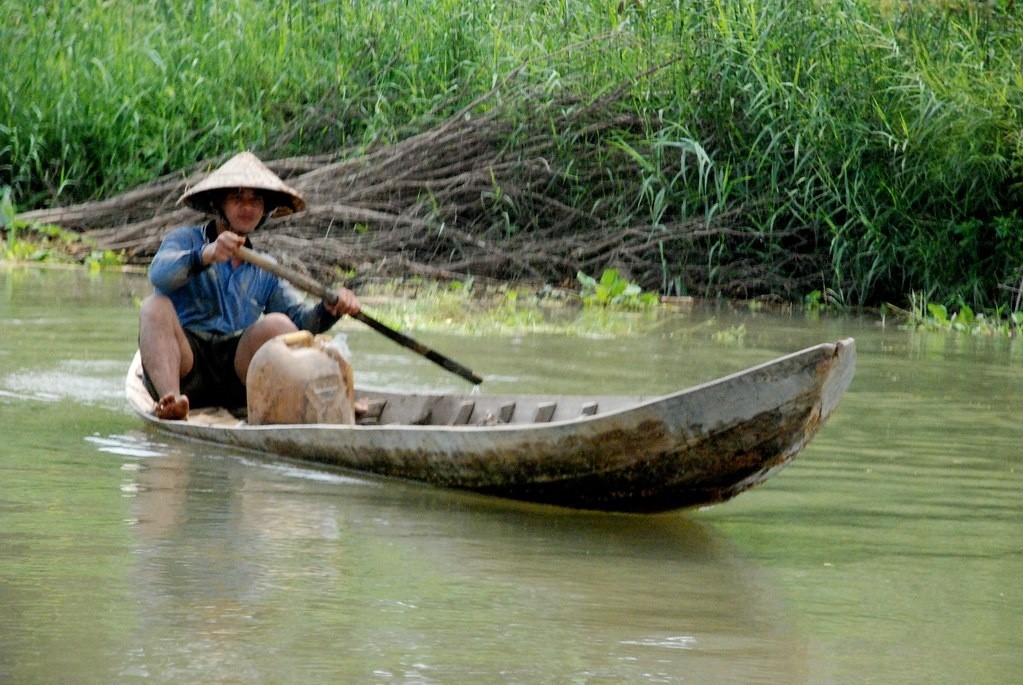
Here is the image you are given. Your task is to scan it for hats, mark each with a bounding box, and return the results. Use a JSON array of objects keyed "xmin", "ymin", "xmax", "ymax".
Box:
[{"xmin": 175, "ymin": 151, "xmax": 305, "ymax": 219}]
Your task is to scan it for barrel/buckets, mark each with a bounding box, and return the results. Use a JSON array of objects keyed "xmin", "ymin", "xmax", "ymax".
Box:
[{"xmin": 245, "ymin": 330, "xmax": 357, "ymax": 424}]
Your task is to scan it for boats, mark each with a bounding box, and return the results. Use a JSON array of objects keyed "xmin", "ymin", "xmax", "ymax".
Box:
[{"xmin": 125, "ymin": 335, "xmax": 860, "ymax": 515}]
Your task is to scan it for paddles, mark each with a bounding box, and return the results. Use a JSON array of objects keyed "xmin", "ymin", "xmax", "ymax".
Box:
[{"xmin": 233, "ymin": 245, "xmax": 482, "ymax": 385}]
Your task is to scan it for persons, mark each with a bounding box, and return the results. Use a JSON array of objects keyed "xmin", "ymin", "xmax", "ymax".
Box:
[{"xmin": 136, "ymin": 149, "xmax": 361, "ymax": 420}]
